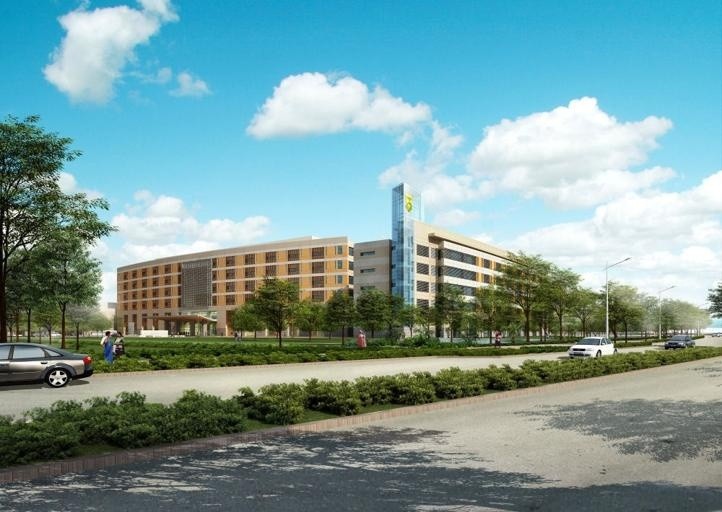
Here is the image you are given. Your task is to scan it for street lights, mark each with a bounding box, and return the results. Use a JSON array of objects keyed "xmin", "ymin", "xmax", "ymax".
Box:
[
  {"xmin": 659, "ymin": 285, "xmax": 674, "ymax": 339},
  {"xmin": 602, "ymin": 256, "xmax": 631, "ymax": 339}
]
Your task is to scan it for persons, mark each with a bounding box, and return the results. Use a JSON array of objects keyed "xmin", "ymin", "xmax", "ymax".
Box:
[
  {"xmin": 494, "ymin": 329, "xmax": 504, "ymax": 349},
  {"xmin": 356, "ymin": 329, "xmax": 367, "ymax": 348},
  {"xmin": 99, "ymin": 330, "xmax": 112, "ymax": 359},
  {"xmin": 234, "ymin": 328, "xmax": 241, "ymax": 342},
  {"xmin": 112, "ymin": 331, "xmax": 126, "ymax": 356}
]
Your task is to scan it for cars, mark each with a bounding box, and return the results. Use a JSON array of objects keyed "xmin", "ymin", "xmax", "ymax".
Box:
[
  {"xmin": 568, "ymin": 335, "xmax": 618, "ymax": 360},
  {"xmin": 665, "ymin": 334, "xmax": 695, "ymax": 349},
  {"xmin": 0, "ymin": 342, "xmax": 94, "ymax": 388}
]
